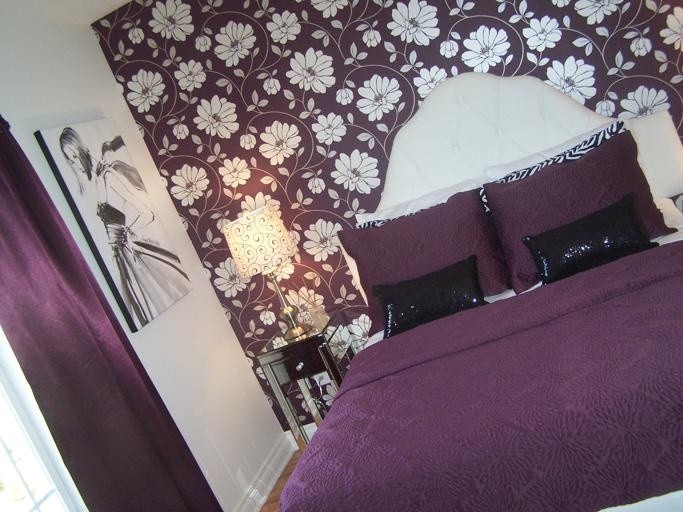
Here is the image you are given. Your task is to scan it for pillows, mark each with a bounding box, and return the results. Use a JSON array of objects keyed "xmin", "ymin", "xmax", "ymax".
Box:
[
  {"xmin": 522, "ymin": 191, "xmax": 660, "ymax": 285},
  {"xmin": 337, "ymin": 190, "xmax": 510, "ymax": 337},
  {"xmin": 372, "ymin": 255, "xmax": 490, "ymax": 339},
  {"xmin": 483, "ymin": 129, "xmax": 678, "ymax": 296}
]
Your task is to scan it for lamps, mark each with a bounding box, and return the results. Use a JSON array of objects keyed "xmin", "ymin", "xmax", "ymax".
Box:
[{"xmin": 220, "ymin": 204, "xmax": 313, "ymax": 341}]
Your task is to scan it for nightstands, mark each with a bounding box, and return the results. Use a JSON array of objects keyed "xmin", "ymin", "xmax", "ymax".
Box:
[{"xmin": 256, "ymin": 309, "xmax": 358, "ymax": 454}]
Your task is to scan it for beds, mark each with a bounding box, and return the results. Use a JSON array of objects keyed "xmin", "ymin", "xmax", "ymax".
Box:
[{"xmin": 277, "ymin": 72, "xmax": 683, "ymax": 512}]
